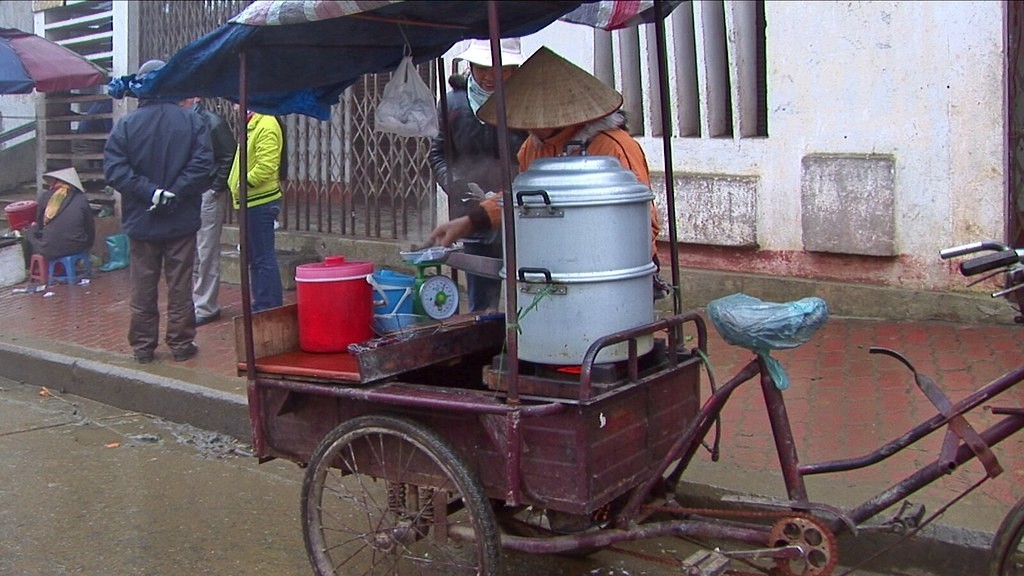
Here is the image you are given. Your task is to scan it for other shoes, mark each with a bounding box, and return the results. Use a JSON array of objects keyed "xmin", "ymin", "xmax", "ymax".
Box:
[
  {"xmin": 173, "ymin": 341, "xmax": 198, "ymax": 362},
  {"xmin": 132, "ymin": 349, "xmax": 154, "ymax": 365},
  {"xmin": 194, "ymin": 309, "xmax": 221, "ymax": 326}
]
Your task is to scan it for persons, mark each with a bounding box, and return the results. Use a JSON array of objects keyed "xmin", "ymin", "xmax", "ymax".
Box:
[
  {"xmin": 426, "ymin": 39, "xmax": 661, "ymax": 316},
  {"xmin": 227, "ymin": 101, "xmax": 288, "ymax": 314},
  {"xmin": 102, "ymin": 60, "xmax": 214, "ymax": 364},
  {"xmin": 22, "ymin": 167, "xmax": 96, "ymax": 269},
  {"xmin": 177, "ymin": 95, "xmax": 237, "ymax": 327},
  {"xmin": 72, "ymin": 99, "xmax": 113, "ymax": 217}
]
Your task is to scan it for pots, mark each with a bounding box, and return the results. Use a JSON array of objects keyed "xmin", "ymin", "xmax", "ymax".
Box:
[{"xmin": 499, "ymin": 140, "xmax": 658, "ymax": 365}]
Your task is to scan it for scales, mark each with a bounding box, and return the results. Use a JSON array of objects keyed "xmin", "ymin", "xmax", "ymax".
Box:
[{"xmin": 398, "ymin": 247, "xmax": 464, "ymax": 325}]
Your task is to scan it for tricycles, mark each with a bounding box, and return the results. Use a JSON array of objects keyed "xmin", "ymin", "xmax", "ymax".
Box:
[{"xmin": 230, "ymin": 0, "xmax": 1024, "ymax": 575}]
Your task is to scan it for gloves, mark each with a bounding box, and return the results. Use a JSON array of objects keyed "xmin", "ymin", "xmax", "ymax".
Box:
[{"xmin": 144, "ymin": 188, "xmax": 180, "ymax": 215}]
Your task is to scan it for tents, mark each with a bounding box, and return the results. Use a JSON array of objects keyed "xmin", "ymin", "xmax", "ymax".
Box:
[{"xmin": 111, "ymin": 0, "xmax": 687, "ymax": 402}]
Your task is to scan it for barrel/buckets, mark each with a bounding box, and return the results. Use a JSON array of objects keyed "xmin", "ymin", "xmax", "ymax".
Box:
[
  {"xmin": 4, "ymin": 201, "xmax": 38, "ymax": 231},
  {"xmin": 371, "ymin": 267, "xmax": 416, "ymax": 336},
  {"xmin": 296, "ymin": 255, "xmax": 389, "ymax": 354}
]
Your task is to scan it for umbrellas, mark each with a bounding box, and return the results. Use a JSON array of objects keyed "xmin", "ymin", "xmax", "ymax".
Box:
[{"xmin": 0, "ymin": 28, "xmax": 108, "ymax": 95}]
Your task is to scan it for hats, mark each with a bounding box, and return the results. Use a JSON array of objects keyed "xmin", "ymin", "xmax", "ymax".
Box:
[
  {"xmin": 476, "ymin": 45, "xmax": 624, "ymax": 130},
  {"xmin": 42, "ymin": 167, "xmax": 85, "ymax": 194},
  {"xmin": 452, "ymin": 36, "xmax": 526, "ymax": 67}
]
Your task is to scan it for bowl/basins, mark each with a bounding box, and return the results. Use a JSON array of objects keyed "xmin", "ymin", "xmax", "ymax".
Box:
[{"xmin": 372, "ymin": 313, "xmax": 427, "ymax": 332}]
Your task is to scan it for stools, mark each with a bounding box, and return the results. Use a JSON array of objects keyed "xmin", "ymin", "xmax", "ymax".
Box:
[{"xmin": 29, "ymin": 249, "xmax": 91, "ymax": 286}]
